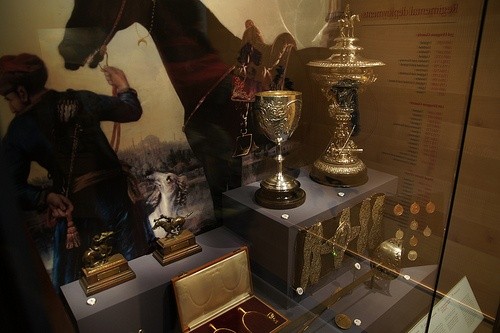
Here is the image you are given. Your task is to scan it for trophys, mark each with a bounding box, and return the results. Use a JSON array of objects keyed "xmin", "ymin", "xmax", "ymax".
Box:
[
  {"xmin": 253, "ymin": 90, "xmax": 306, "ymax": 209},
  {"xmin": 306, "ymin": 4, "xmax": 386, "ymax": 187}
]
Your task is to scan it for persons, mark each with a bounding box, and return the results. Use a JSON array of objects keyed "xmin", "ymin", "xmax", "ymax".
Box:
[
  {"xmin": 90, "ymin": 234, "xmax": 107, "ymax": 252},
  {"xmin": 153, "ymin": 215, "xmax": 177, "ymax": 223}
]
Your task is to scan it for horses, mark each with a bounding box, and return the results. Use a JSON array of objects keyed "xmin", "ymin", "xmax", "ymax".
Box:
[{"xmin": 57, "ymin": 0, "xmax": 360, "ymax": 227}]
